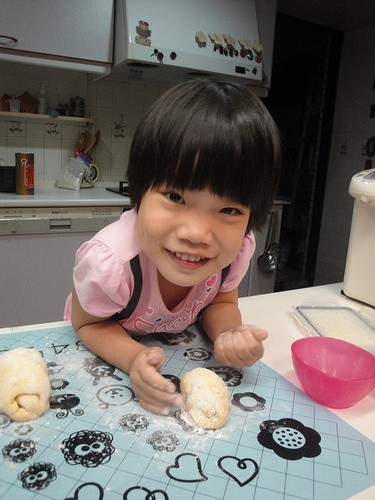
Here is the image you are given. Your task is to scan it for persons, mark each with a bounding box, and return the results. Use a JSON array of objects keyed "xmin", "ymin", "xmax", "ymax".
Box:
[{"xmin": 63, "ymin": 71, "xmax": 280, "ymax": 414}]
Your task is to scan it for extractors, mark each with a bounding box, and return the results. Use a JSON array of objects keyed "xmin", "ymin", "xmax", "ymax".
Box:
[{"xmin": 103, "ymin": 0, "xmax": 263, "ymax": 94}]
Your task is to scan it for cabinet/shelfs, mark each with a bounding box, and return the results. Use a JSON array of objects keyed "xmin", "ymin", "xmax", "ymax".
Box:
[
  {"xmin": 0, "ymin": 203, "xmax": 128, "ymax": 330},
  {"xmin": 1, "ymin": 0, "xmax": 115, "ymax": 81}
]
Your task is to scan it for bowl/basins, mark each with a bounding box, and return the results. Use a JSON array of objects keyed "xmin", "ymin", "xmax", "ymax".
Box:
[{"xmin": 291, "ymin": 336, "xmax": 375, "ymax": 411}]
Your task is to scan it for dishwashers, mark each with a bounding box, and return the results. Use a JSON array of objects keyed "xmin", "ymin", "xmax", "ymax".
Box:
[{"xmin": 0, "ymin": 206, "xmax": 130, "ymax": 330}]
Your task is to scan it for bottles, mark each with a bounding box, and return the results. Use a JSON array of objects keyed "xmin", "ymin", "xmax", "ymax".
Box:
[{"xmin": 38, "ymin": 80, "xmax": 49, "ymax": 114}]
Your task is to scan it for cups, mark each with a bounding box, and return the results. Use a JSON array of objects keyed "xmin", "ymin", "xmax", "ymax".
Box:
[
  {"xmin": 9, "ymin": 99, "xmax": 21, "ymax": 112},
  {"xmin": 70, "ymin": 158, "xmax": 100, "ymax": 188}
]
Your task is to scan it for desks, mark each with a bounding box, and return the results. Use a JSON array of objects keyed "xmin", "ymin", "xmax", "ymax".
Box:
[{"xmin": 1, "ymin": 279, "xmax": 375, "ymax": 500}]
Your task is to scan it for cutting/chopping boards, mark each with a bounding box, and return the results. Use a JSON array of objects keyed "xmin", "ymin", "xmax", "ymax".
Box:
[{"xmin": 1, "ymin": 320, "xmax": 375, "ymax": 500}]
